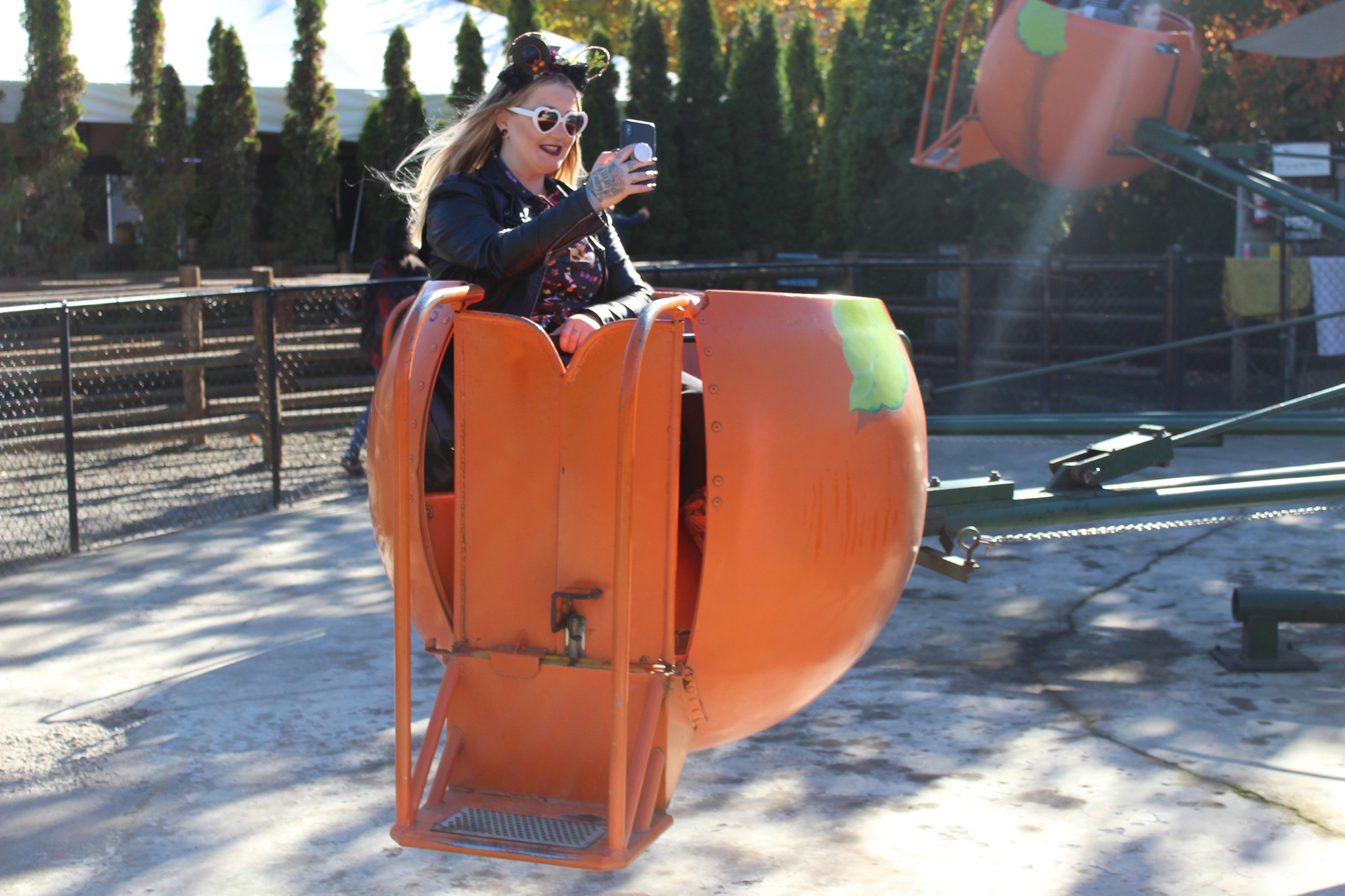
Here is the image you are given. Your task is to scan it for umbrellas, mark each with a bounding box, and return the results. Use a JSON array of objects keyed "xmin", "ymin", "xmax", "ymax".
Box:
[{"xmin": 1230, "ymin": 0, "xmax": 1345, "ymax": 60}]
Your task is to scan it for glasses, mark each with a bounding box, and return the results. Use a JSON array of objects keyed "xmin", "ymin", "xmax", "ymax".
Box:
[{"xmin": 506, "ymin": 105, "xmax": 588, "ymax": 138}]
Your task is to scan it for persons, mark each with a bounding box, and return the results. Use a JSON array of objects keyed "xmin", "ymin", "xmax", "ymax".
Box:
[
  {"xmin": 1054, "ymin": 0, "xmax": 1161, "ymax": 30},
  {"xmin": 386, "ymin": 32, "xmax": 707, "ymax": 555},
  {"xmin": 343, "ymin": 253, "xmax": 428, "ymax": 478}
]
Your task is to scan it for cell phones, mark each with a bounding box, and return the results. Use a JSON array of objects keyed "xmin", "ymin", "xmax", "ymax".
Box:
[{"xmin": 621, "ymin": 118, "xmax": 657, "ymax": 189}]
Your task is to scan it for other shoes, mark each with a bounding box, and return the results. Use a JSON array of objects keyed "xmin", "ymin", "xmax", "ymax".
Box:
[{"xmin": 340, "ymin": 456, "xmax": 366, "ymax": 476}]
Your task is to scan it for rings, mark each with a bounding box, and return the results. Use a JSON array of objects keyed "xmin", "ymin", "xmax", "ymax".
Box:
[{"xmin": 630, "ymin": 172, "xmax": 636, "ymax": 184}]
